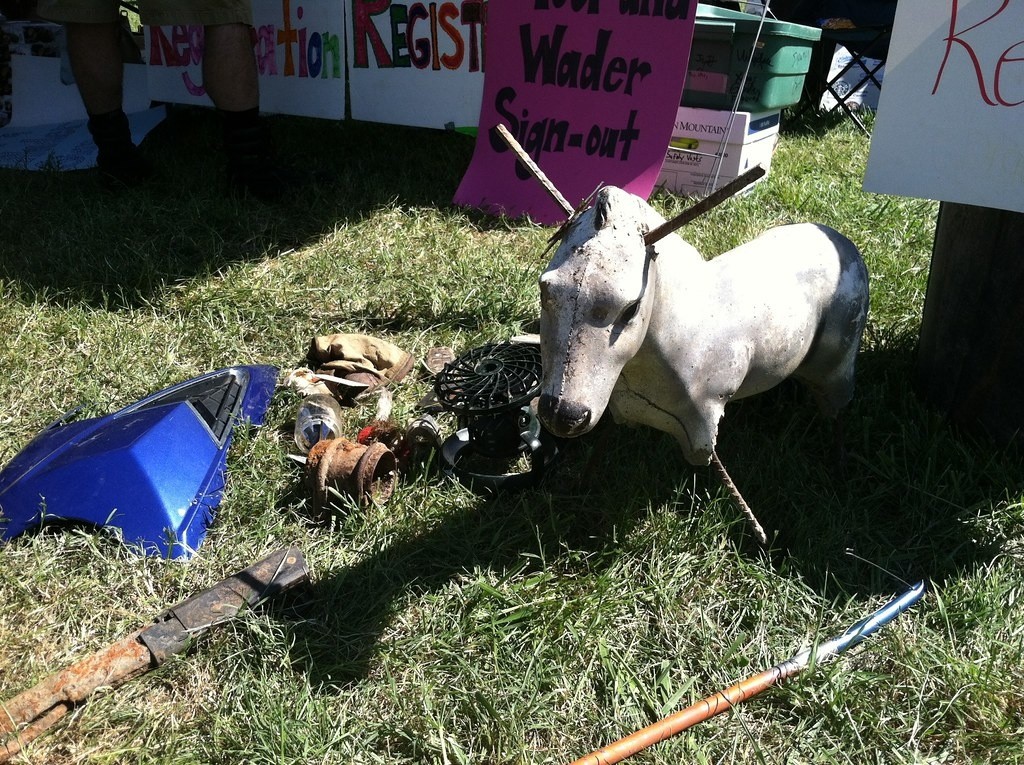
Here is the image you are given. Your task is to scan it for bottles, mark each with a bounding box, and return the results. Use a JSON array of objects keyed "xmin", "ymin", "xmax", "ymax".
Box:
[{"xmin": 295, "ymin": 392, "xmax": 341, "ymax": 456}]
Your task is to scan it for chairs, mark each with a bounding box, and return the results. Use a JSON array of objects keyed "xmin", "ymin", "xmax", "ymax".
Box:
[{"xmin": 783, "ymin": 0, "xmax": 898, "ymax": 133}]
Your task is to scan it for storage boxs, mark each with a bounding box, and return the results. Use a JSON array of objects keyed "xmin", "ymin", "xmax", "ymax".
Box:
[{"xmin": 651, "ymin": 3, "xmax": 822, "ymax": 204}]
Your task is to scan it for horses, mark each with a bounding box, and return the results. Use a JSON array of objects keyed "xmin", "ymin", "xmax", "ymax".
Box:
[{"xmin": 537, "ymin": 186, "xmax": 870, "ymax": 469}]
